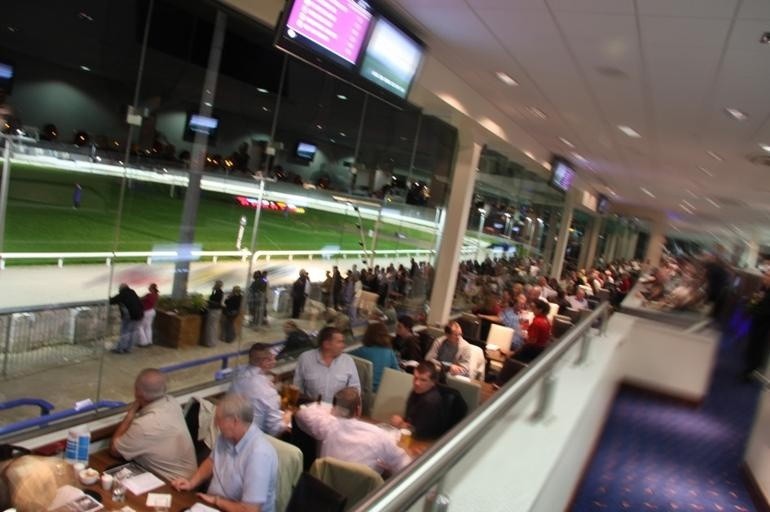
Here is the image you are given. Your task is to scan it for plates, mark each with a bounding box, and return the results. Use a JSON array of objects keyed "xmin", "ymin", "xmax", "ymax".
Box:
[{"xmin": 104, "ymin": 463, "xmax": 146, "ymax": 481}]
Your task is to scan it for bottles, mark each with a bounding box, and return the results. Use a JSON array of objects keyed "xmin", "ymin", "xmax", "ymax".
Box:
[
  {"xmin": 79, "ymin": 424, "xmax": 92, "ymax": 467},
  {"xmin": 67, "ymin": 430, "xmax": 77, "ymax": 463}
]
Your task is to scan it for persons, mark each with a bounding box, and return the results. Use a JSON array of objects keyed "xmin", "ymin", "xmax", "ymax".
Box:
[
  {"xmin": 319, "ymin": 271, "xmax": 333, "ymax": 312},
  {"xmin": 169, "ymin": 392, "xmax": 278, "ymax": 510},
  {"xmin": 109, "ymin": 283, "xmax": 145, "ymax": 354},
  {"xmin": 331, "ymin": 266, "xmax": 342, "ymax": 311},
  {"xmin": 360, "ymin": 252, "xmax": 555, "ymax": 298},
  {"xmin": 373, "ymin": 298, "xmax": 398, "ymax": 333},
  {"xmin": 348, "ymin": 275, "xmax": 363, "ymax": 322},
  {"xmin": 425, "ymin": 321, "xmax": 473, "ymax": 380},
  {"xmin": 292, "ymin": 327, "xmax": 362, "ymax": 404},
  {"xmin": 390, "ymin": 315, "xmax": 421, "ymax": 364},
  {"xmin": 492, "ymin": 298, "xmax": 550, "ymax": 390},
  {"xmin": 220, "ymin": 285, "xmax": 243, "ymax": 343},
  {"xmin": 111, "ymin": 367, "xmax": 197, "ymax": 483},
  {"xmin": 348, "ymin": 324, "xmax": 400, "ymax": 395},
  {"xmin": 295, "ymin": 386, "xmax": 404, "ymax": 473},
  {"xmin": 498, "ymin": 298, "xmax": 524, "ymax": 352},
  {"xmin": 227, "ymin": 343, "xmax": 293, "ymax": 438},
  {"xmin": 250, "ymin": 271, "xmax": 267, "ymax": 327},
  {"xmin": 343, "ymin": 268, "xmax": 354, "ymax": 284},
  {"xmin": 135, "ymin": 283, "xmax": 159, "ymax": 348},
  {"xmin": 199, "ymin": 280, "xmax": 224, "ymax": 347},
  {"xmin": 390, "ymin": 360, "xmax": 449, "ymax": 459},
  {"xmin": 559, "ymin": 247, "xmax": 644, "ymax": 306},
  {"xmin": 549, "ymin": 291, "xmax": 571, "ymax": 316},
  {"xmin": 290, "ymin": 269, "xmax": 308, "ymax": 319},
  {"xmin": 351, "ymin": 264, "xmax": 359, "ymax": 283}
]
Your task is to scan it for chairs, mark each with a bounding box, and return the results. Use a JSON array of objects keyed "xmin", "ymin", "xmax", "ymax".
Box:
[
  {"xmin": 253, "ymin": 433, "xmax": 303, "ymax": 512},
  {"xmin": 346, "ymin": 354, "xmax": 374, "ymax": 415},
  {"xmin": 452, "ymin": 311, "xmax": 483, "ymax": 339},
  {"xmin": 371, "ymin": 366, "xmax": 420, "ymax": 422},
  {"xmin": 597, "ymin": 288, "xmax": 609, "ymax": 302},
  {"xmin": 489, "ymin": 324, "xmax": 514, "ymax": 349},
  {"xmin": 586, "ymin": 300, "xmax": 598, "ymax": 309},
  {"xmin": 306, "ymin": 456, "xmax": 385, "ymax": 503},
  {"xmin": 443, "ymin": 374, "xmax": 484, "ymax": 411},
  {"xmin": 183, "ymin": 397, "xmax": 227, "ymax": 467},
  {"xmin": 553, "ymin": 319, "xmax": 572, "ymax": 340},
  {"xmin": 564, "ymin": 306, "xmax": 578, "ymax": 320},
  {"xmin": 543, "ymin": 302, "xmax": 559, "ymax": 318},
  {"xmin": 552, "ymin": 314, "xmax": 573, "ymax": 333}
]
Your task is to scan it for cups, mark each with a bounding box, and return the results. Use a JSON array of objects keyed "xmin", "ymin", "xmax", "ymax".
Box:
[
  {"xmin": 112, "ymin": 472, "xmax": 125, "ymax": 503},
  {"xmin": 155, "ymin": 497, "xmax": 167, "ymax": 512}
]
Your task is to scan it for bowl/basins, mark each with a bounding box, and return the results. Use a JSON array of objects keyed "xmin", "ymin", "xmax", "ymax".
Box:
[{"xmin": 80, "ymin": 470, "xmax": 98, "ymax": 485}]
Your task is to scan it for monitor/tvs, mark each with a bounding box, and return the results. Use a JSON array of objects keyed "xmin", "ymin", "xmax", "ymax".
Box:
[
  {"xmin": 597, "ymin": 195, "xmax": 608, "ymax": 214},
  {"xmin": 547, "ymin": 159, "xmax": 576, "ymax": 194},
  {"xmin": 352, "ymin": 9, "xmax": 431, "ymax": 107},
  {"xmin": 279, "ymin": 0, "xmax": 379, "ymax": 76}
]
